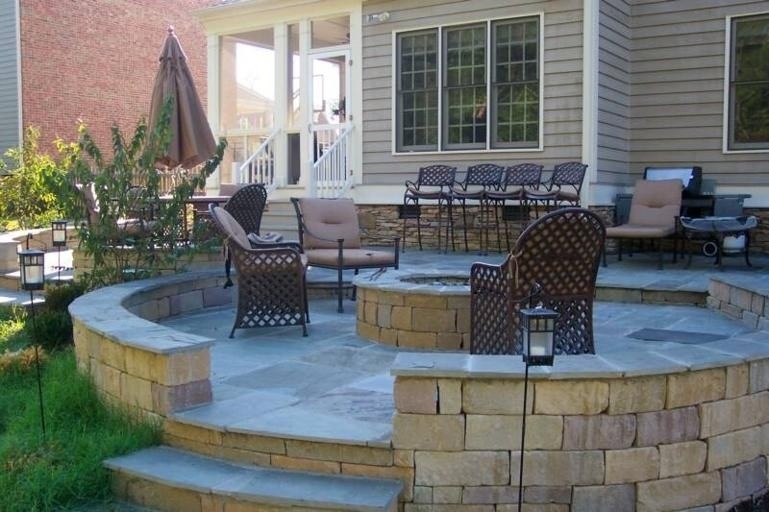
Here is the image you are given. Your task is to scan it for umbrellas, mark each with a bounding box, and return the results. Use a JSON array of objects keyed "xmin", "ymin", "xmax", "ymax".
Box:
[{"xmin": 141, "ymin": 25, "xmax": 221, "ymax": 245}]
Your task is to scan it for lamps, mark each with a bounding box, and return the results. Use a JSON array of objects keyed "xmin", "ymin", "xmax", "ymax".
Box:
[
  {"xmin": 365, "ymin": 11, "xmax": 390, "ymax": 25},
  {"xmin": 514, "ymin": 282, "xmax": 559, "ymax": 510},
  {"xmin": 17, "ymin": 218, "xmax": 67, "ymax": 450}
]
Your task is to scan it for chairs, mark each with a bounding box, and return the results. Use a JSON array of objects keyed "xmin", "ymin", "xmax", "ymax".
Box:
[
  {"xmin": 77, "ymin": 181, "xmax": 164, "ymax": 268},
  {"xmin": 402, "ymin": 164, "xmax": 460, "ymax": 253},
  {"xmin": 206, "ymin": 201, "xmax": 313, "ymax": 339},
  {"xmin": 483, "ymin": 163, "xmax": 544, "ymax": 258},
  {"xmin": 248, "ymin": 132, "xmax": 323, "ymax": 183},
  {"xmin": 523, "ymin": 161, "xmax": 588, "ymax": 236},
  {"xmin": 190, "ymin": 183, "xmax": 269, "ymax": 256},
  {"xmin": 601, "ymin": 177, "xmax": 685, "ymax": 267},
  {"xmin": 674, "ymin": 194, "xmax": 756, "ymax": 270},
  {"xmin": 291, "ymin": 196, "xmax": 401, "ymax": 314},
  {"xmin": 447, "ymin": 161, "xmax": 503, "ymax": 257},
  {"xmin": 467, "ymin": 207, "xmax": 606, "ymax": 356}
]
